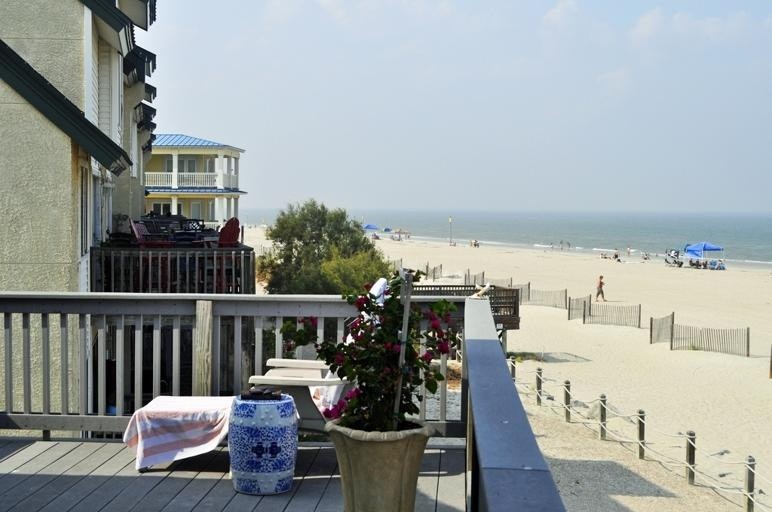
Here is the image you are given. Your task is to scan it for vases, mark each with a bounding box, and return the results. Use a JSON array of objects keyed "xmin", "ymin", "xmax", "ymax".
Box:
[{"xmin": 324, "ymin": 418, "xmax": 436, "ymax": 512}]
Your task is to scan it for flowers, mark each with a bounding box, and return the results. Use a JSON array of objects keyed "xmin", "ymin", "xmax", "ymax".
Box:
[{"xmin": 315, "ymin": 266, "xmax": 455, "ymax": 430}]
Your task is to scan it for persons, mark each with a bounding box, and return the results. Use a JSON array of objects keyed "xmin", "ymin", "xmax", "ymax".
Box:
[
  {"xmin": 474, "ymin": 240, "xmax": 479, "ymax": 248},
  {"xmin": 595, "ymin": 276, "xmax": 607, "ymax": 301},
  {"xmin": 614, "ymin": 248, "xmax": 723, "ymax": 270}
]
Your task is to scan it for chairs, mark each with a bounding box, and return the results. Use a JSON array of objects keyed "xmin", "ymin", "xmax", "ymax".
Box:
[
  {"xmin": 123, "ymin": 332, "xmax": 360, "ymax": 474},
  {"xmin": 665, "ymin": 258, "xmax": 724, "ymax": 270},
  {"xmin": 127, "ymin": 215, "xmax": 240, "ymax": 284}
]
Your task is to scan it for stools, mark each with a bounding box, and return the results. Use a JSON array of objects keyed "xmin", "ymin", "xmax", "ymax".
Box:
[{"xmin": 228, "ymin": 394, "xmax": 294, "ymax": 495}]
